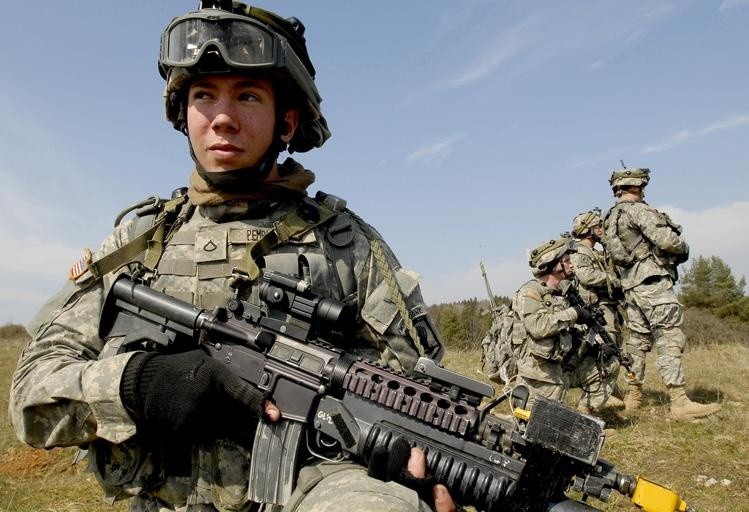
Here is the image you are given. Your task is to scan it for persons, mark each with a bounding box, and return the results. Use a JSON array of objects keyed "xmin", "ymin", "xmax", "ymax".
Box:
[
  {"xmin": 603, "ymin": 167, "xmax": 723, "ymax": 421},
  {"xmin": 569, "ymin": 207, "xmax": 629, "ymax": 424},
  {"xmin": 10, "ymin": 0, "xmax": 459, "ymax": 512},
  {"xmin": 509, "ymin": 241, "xmax": 609, "ymax": 409}
]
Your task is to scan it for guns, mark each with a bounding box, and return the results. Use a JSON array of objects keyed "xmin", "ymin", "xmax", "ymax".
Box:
[
  {"xmin": 562, "ymin": 283, "xmax": 637, "ymax": 381},
  {"xmin": 97, "ymin": 273, "xmax": 688, "ymax": 512}
]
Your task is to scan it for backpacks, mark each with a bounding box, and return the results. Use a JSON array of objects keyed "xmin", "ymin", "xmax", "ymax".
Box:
[
  {"xmin": 481, "ymin": 304, "xmax": 520, "ymax": 385},
  {"xmin": 605, "ymin": 210, "xmax": 633, "ymax": 268}
]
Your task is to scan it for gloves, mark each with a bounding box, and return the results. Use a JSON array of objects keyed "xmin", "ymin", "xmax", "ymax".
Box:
[
  {"xmin": 574, "ymin": 306, "xmax": 592, "ymax": 325},
  {"xmin": 597, "ymin": 344, "xmax": 615, "ymax": 361},
  {"xmin": 367, "ymin": 436, "xmax": 465, "ymax": 511},
  {"xmin": 119, "ymin": 349, "xmax": 278, "ymax": 428}
]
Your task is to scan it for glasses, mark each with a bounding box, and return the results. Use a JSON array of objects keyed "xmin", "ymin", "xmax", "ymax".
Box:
[
  {"xmin": 556, "ymin": 240, "xmax": 578, "ymax": 258},
  {"xmin": 159, "ymin": 11, "xmax": 277, "ymax": 67}
]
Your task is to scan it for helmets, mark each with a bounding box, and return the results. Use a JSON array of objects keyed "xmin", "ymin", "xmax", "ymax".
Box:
[
  {"xmin": 164, "ymin": 0, "xmax": 331, "ymax": 152},
  {"xmin": 610, "ymin": 168, "xmax": 650, "ymax": 186},
  {"xmin": 571, "ymin": 207, "xmax": 601, "ymax": 237},
  {"xmin": 529, "ymin": 238, "xmax": 571, "ymax": 278}
]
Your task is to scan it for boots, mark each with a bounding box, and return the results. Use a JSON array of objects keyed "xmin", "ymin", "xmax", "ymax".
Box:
[
  {"xmin": 577, "ymin": 384, "xmax": 649, "ymax": 415},
  {"xmin": 669, "ymin": 387, "xmax": 721, "ymax": 419}
]
